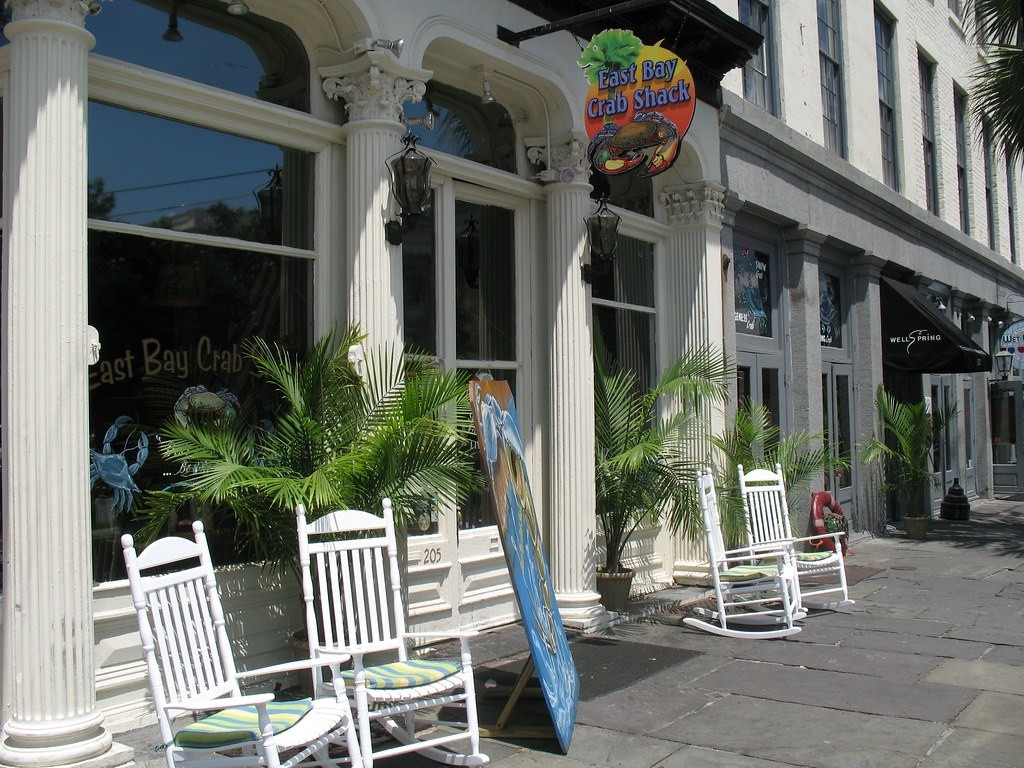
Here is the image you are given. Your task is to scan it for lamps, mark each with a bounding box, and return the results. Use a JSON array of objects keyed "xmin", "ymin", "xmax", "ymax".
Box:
[
  {"xmin": 963, "ymin": 312, "xmax": 975, "ymax": 321},
  {"xmin": 988, "ymin": 347, "xmax": 1013, "ymax": 382},
  {"xmin": 998, "ymin": 320, "xmax": 1004, "ymax": 325},
  {"xmin": 987, "ymin": 315, "xmax": 992, "ymax": 322},
  {"xmin": 583, "ymin": 192, "xmax": 623, "ymax": 284},
  {"xmin": 935, "ymin": 296, "xmax": 946, "ymax": 311},
  {"xmin": 385, "ymin": 127, "xmax": 437, "ymax": 246}
]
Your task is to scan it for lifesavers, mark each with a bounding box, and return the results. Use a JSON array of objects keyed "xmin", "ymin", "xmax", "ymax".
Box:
[{"xmin": 812, "ymin": 491, "xmax": 849, "ymax": 555}]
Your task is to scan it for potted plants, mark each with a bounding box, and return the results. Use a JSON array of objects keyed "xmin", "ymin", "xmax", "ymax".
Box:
[
  {"xmin": 591, "ymin": 340, "xmax": 743, "ymax": 610},
  {"xmin": 673, "ymin": 394, "xmax": 855, "ymax": 592},
  {"xmin": 859, "ymin": 384, "xmax": 964, "ymax": 539},
  {"xmin": 134, "ymin": 314, "xmax": 489, "ymax": 697}
]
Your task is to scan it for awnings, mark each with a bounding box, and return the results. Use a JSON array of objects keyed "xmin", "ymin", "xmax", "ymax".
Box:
[
  {"xmin": 507, "ymin": 0, "xmax": 764, "ymax": 110},
  {"xmin": 880, "ymin": 276, "xmax": 994, "ymax": 374}
]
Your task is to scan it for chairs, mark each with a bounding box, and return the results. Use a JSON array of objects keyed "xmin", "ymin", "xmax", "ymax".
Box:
[
  {"xmin": 120, "ymin": 521, "xmax": 364, "ymax": 768},
  {"xmin": 683, "ymin": 467, "xmax": 806, "ymax": 639},
  {"xmin": 734, "ymin": 462, "xmax": 855, "ymax": 621},
  {"xmin": 295, "ymin": 498, "xmax": 489, "ymax": 768}
]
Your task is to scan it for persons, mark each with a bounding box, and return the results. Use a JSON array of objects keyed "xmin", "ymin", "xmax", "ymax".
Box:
[{"xmin": 173, "ymin": 385, "xmax": 254, "ymax": 533}]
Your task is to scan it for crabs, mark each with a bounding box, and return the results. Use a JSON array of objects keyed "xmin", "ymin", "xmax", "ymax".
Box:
[{"xmin": 587, "ymin": 111, "xmax": 682, "ymax": 178}]
